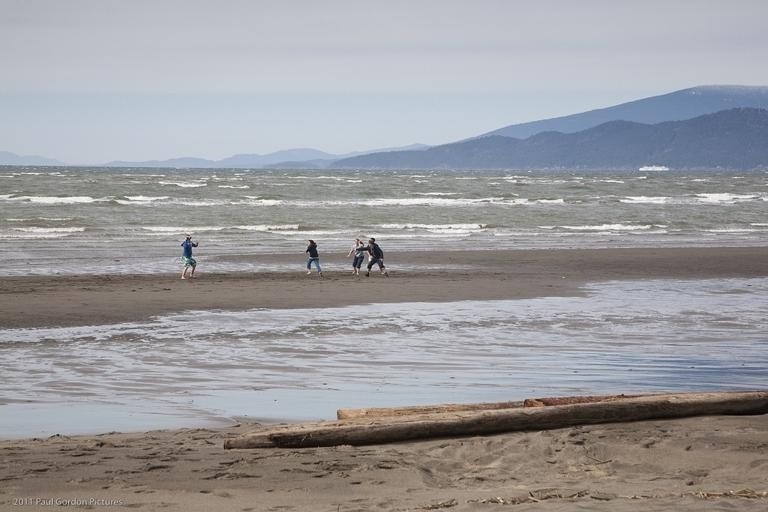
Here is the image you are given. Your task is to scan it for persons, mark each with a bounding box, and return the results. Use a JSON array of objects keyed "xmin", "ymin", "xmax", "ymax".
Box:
[
  {"xmin": 180, "ymin": 235, "xmax": 198, "ymax": 280},
  {"xmin": 347, "ymin": 239, "xmax": 364, "ymax": 275},
  {"xmin": 306, "ymin": 240, "xmax": 322, "ymax": 275},
  {"xmin": 356, "ymin": 238, "xmax": 386, "ymax": 277}
]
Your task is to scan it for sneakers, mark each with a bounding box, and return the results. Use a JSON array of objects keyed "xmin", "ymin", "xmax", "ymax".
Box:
[
  {"xmin": 365, "ymin": 272, "xmax": 369, "ymax": 276},
  {"xmin": 384, "ymin": 272, "xmax": 388, "ymax": 276}
]
